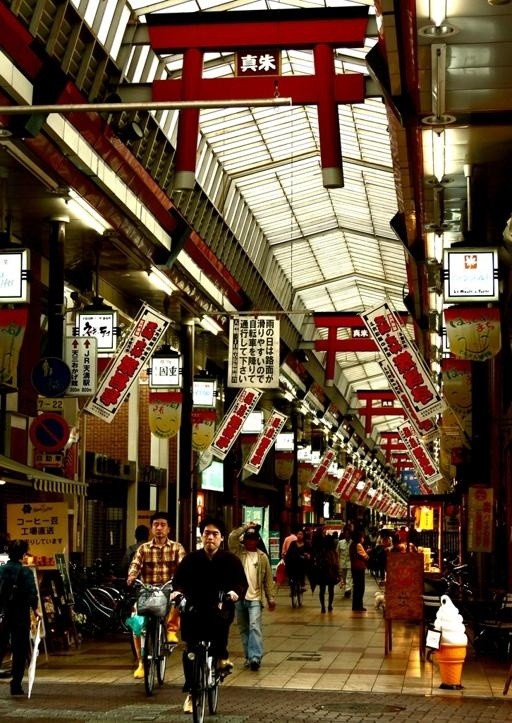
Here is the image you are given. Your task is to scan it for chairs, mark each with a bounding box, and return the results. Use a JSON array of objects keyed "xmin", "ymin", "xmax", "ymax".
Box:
[{"xmin": 474, "ymin": 591, "xmax": 512, "ymax": 661}]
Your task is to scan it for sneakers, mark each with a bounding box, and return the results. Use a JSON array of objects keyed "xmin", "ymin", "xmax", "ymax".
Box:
[
  {"xmin": 183, "ymin": 694, "xmax": 194, "ymax": 714},
  {"xmin": 134, "ymin": 659, "xmax": 145, "ymax": 679},
  {"xmin": 216, "ymin": 659, "xmax": 233, "ymax": 669},
  {"xmin": 167, "ymin": 632, "xmax": 178, "ymax": 642}
]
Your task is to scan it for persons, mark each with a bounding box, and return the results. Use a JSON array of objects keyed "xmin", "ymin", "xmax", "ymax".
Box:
[
  {"xmin": 280, "ymin": 518, "xmax": 419, "ymax": 612},
  {"xmin": 0, "ymin": 535, "xmax": 42, "ymax": 696},
  {"xmin": 116, "ymin": 524, "xmax": 150, "ymax": 576},
  {"xmin": 168, "ymin": 517, "xmax": 250, "ymax": 714},
  {"xmin": 227, "ymin": 519, "xmax": 277, "ymax": 670},
  {"xmin": 127, "ymin": 511, "xmax": 186, "ymax": 677}
]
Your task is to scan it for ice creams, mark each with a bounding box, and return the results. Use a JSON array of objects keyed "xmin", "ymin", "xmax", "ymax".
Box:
[{"xmin": 431, "ymin": 594, "xmax": 470, "ymax": 686}]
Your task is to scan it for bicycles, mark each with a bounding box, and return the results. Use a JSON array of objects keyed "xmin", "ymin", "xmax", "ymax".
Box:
[
  {"xmin": 182, "ymin": 592, "xmax": 233, "ymax": 722},
  {"xmin": 127, "ymin": 577, "xmax": 173, "ymax": 696},
  {"xmin": 286, "ymin": 555, "xmax": 307, "ymax": 608},
  {"xmin": 67, "ymin": 559, "xmax": 136, "ymax": 636}
]
